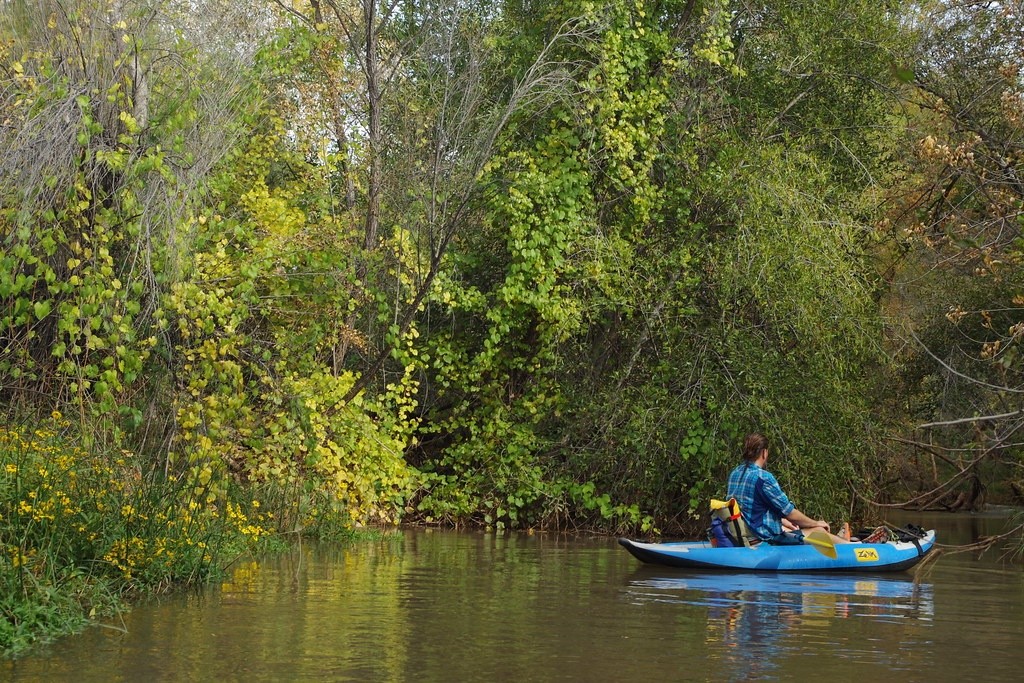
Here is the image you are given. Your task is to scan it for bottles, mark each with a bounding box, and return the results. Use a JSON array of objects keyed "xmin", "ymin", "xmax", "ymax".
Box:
[{"xmin": 710, "ymin": 538, "xmax": 718, "ymax": 548}]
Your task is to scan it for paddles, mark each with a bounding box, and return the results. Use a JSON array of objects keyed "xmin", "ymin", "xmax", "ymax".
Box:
[{"xmin": 781, "ymin": 526, "xmax": 839, "ymax": 560}]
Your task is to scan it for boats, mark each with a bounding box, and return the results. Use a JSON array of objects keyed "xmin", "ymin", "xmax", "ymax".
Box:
[
  {"xmin": 617, "ymin": 525, "xmax": 938, "ymax": 573},
  {"xmin": 626, "ymin": 566, "xmax": 936, "ymax": 628}
]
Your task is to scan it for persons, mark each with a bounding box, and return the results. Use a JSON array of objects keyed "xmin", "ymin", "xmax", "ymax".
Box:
[{"xmin": 726, "ymin": 433, "xmax": 890, "ymax": 545}]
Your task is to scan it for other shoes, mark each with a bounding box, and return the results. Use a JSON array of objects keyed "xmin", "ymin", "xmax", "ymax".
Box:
[
  {"xmin": 837, "ymin": 523, "xmax": 850, "ymax": 541},
  {"xmin": 863, "ymin": 526, "xmax": 890, "ymax": 543}
]
